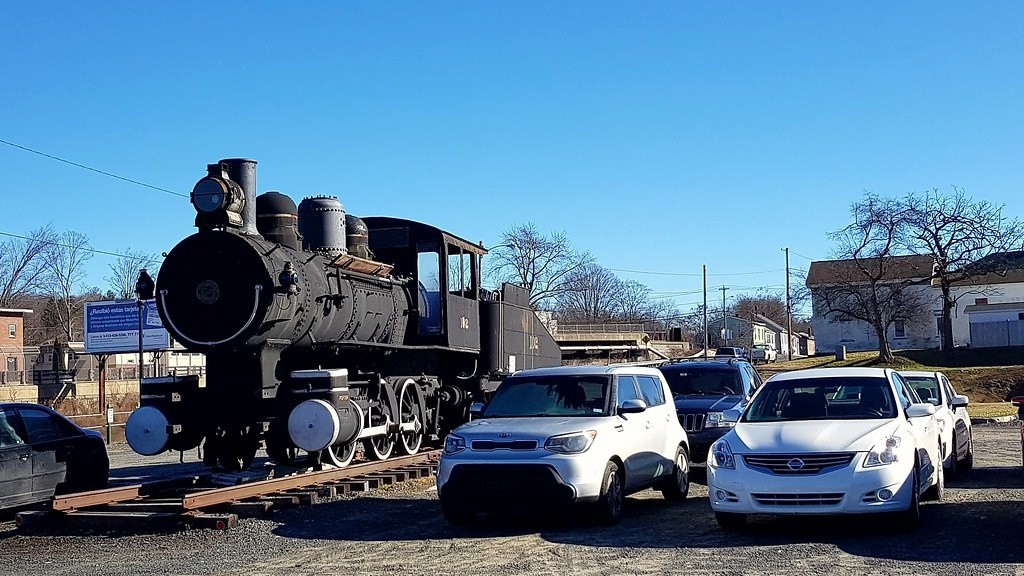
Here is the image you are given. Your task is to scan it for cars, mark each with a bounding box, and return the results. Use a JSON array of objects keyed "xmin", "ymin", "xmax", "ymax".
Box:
[
  {"xmin": 898, "ymin": 372, "xmax": 974, "ymax": 476},
  {"xmin": 714, "ymin": 346, "xmax": 748, "ymax": 363},
  {"xmin": 0, "ymin": 402, "xmax": 109, "ymax": 516},
  {"xmin": 707, "ymin": 368, "xmax": 946, "ymax": 528}
]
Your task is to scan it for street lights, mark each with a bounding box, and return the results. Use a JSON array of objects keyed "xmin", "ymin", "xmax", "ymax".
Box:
[{"xmin": 721, "ymin": 284, "xmax": 734, "ymax": 344}]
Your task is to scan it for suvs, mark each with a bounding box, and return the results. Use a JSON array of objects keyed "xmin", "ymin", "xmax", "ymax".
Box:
[
  {"xmin": 655, "ymin": 356, "xmax": 764, "ymax": 454},
  {"xmin": 437, "ymin": 364, "xmax": 688, "ymax": 520}
]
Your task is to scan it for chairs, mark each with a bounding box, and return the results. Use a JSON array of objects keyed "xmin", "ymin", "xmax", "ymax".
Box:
[
  {"xmin": 790, "ymin": 386, "xmax": 890, "ymax": 417},
  {"xmin": 677, "ymin": 375, "xmax": 741, "ymax": 396},
  {"xmin": 916, "ymin": 387, "xmax": 938, "ymax": 405}
]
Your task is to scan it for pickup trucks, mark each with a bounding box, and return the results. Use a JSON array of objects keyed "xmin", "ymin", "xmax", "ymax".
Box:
[{"xmin": 747, "ymin": 343, "xmax": 777, "ymax": 364}]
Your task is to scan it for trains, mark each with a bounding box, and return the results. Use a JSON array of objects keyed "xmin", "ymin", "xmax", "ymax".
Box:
[{"xmin": 125, "ymin": 159, "xmax": 563, "ymax": 480}]
